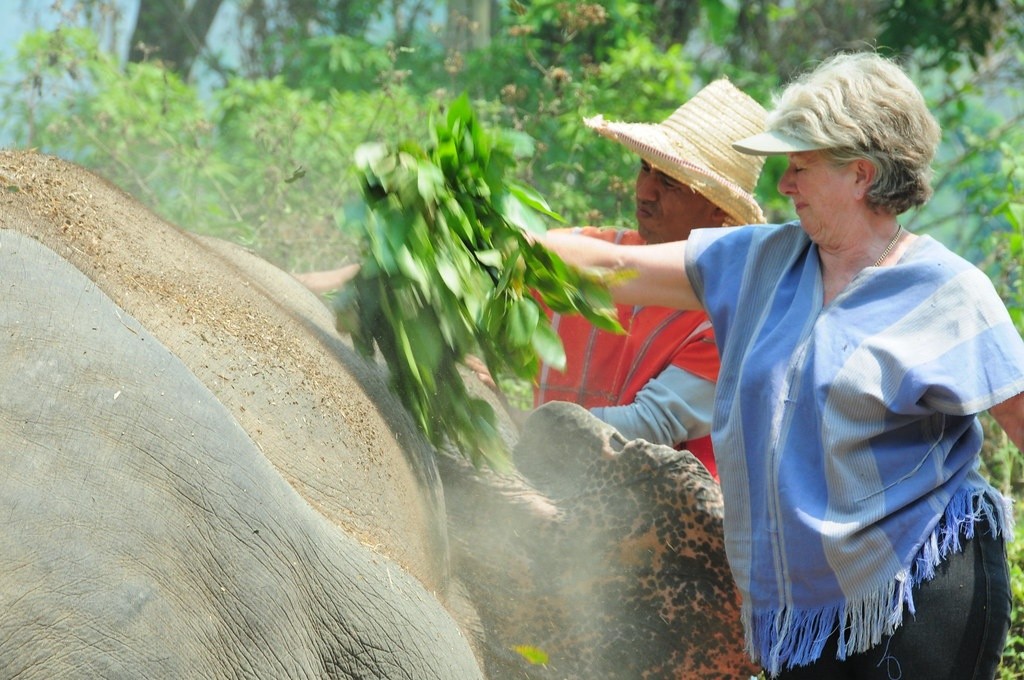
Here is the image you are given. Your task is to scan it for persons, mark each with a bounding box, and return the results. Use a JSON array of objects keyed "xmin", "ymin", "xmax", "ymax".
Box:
[
  {"xmin": 528, "ymin": 52, "xmax": 1024, "ymax": 680},
  {"xmin": 289, "ymin": 79, "xmax": 776, "ymax": 484}
]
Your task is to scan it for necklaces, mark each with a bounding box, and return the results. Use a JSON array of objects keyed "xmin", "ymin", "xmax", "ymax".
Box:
[{"xmin": 873, "ymin": 224, "xmax": 903, "ymax": 267}]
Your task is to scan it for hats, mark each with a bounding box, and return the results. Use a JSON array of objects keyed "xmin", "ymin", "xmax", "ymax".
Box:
[
  {"xmin": 731, "ymin": 129, "xmax": 834, "ymax": 155},
  {"xmin": 582, "ymin": 75, "xmax": 772, "ymax": 226}
]
